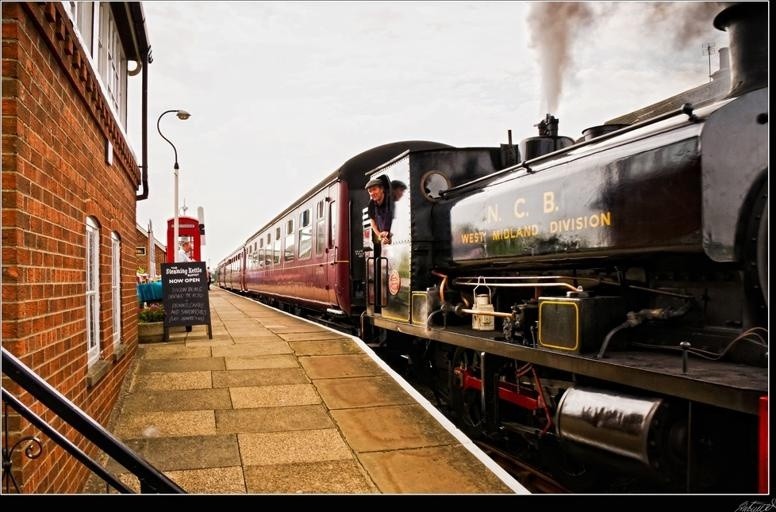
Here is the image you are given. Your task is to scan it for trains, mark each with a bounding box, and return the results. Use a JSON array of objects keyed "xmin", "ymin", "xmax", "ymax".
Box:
[{"xmin": 212, "ymin": 2, "xmax": 774, "ymax": 495}]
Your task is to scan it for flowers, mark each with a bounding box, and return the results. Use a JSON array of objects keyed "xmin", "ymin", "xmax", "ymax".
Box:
[{"xmin": 140, "ymin": 303, "xmax": 164, "ymax": 322}]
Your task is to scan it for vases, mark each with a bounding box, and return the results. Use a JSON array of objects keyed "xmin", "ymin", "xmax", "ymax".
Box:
[{"xmin": 138, "ymin": 322, "xmax": 164, "ymax": 344}]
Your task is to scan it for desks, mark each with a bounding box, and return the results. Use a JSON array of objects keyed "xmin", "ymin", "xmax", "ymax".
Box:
[{"xmin": 137, "ymin": 282, "xmax": 163, "ymax": 303}]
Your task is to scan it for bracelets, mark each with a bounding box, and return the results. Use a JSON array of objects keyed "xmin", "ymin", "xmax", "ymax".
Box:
[{"xmin": 377, "ymin": 234, "xmax": 383, "ymax": 241}]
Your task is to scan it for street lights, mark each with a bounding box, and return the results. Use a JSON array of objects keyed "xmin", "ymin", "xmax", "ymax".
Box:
[{"xmin": 156, "ymin": 106, "xmax": 192, "ymax": 262}]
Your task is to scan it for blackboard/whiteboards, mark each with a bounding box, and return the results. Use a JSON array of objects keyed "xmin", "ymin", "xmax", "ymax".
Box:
[{"xmin": 161, "ymin": 261, "xmax": 210, "ymax": 326}]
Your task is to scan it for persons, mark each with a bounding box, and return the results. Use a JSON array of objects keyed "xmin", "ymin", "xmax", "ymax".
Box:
[
  {"xmin": 366, "ymin": 178, "xmax": 393, "ymax": 244},
  {"xmin": 206, "ymin": 265, "xmax": 211, "ymax": 291},
  {"xmin": 389, "ymin": 180, "xmax": 408, "ymax": 238}
]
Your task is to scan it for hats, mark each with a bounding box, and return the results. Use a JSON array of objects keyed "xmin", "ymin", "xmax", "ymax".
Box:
[
  {"xmin": 365, "ymin": 178, "xmax": 385, "ymax": 190},
  {"xmin": 391, "ymin": 181, "xmax": 407, "ymax": 190}
]
